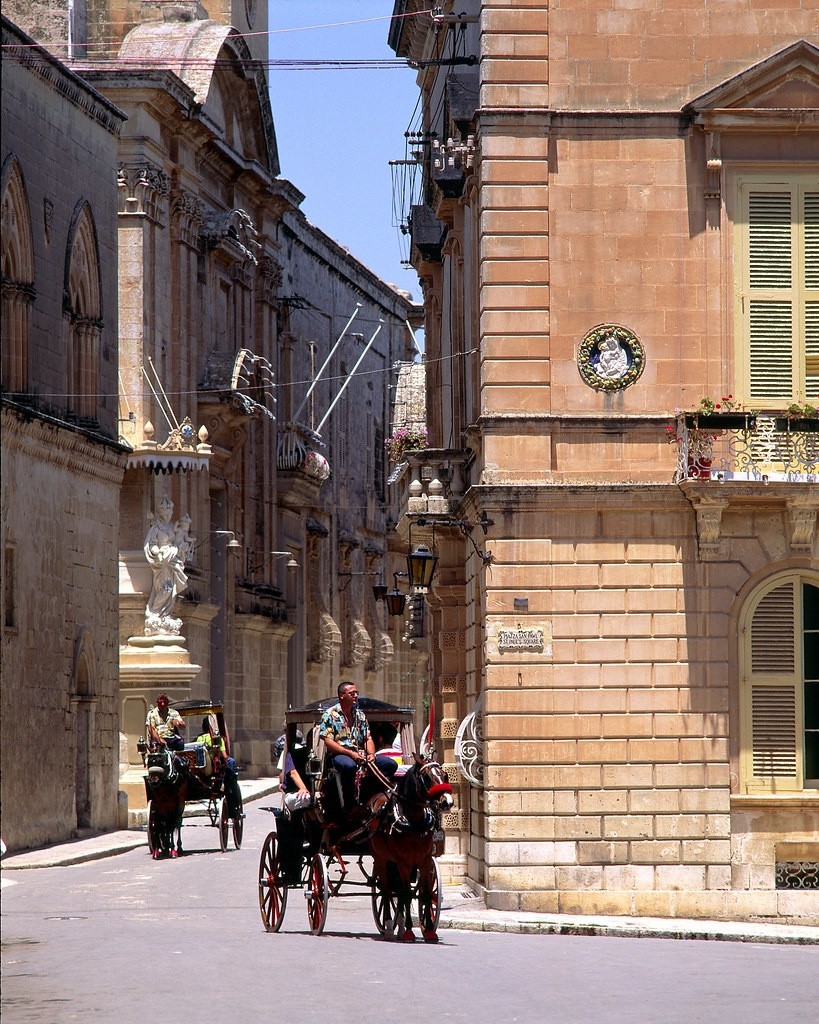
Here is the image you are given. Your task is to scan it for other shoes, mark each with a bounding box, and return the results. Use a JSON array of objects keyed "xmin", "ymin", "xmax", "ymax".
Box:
[
  {"xmin": 362, "ymin": 802, "xmax": 372, "ymax": 819},
  {"xmin": 345, "ymin": 804, "xmax": 361, "ymax": 823}
]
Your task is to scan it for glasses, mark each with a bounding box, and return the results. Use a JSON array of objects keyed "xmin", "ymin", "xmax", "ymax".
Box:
[{"xmin": 340, "ymin": 690, "xmax": 360, "ymax": 696}]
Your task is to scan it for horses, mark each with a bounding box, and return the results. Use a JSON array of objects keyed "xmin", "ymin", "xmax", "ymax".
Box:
[
  {"xmin": 143, "ymin": 742, "xmax": 187, "ymax": 860},
  {"xmin": 366, "ymin": 752, "xmax": 455, "ymax": 944}
]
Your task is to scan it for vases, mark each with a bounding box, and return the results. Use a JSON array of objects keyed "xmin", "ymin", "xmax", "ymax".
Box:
[{"xmin": 683, "ymin": 456, "xmax": 713, "ymax": 480}]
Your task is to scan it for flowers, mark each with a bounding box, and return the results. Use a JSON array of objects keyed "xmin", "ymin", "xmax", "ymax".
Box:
[{"xmin": 667, "ymin": 394, "xmax": 742, "ymax": 459}]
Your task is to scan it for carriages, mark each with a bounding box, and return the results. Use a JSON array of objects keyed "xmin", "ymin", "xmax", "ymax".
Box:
[
  {"xmin": 258, "ymin": 697, "xmax": 455, "ymax": 944},
  {"xmin": 135, "ymin": 700, "xmax": 246, "ymax": 861}
]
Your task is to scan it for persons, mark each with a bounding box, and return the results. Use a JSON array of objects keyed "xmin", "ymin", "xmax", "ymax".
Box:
[
  {"xmin": 318, "ymin": 682, "xmax": 398, "ymax": 821},
  {"xmin": 370, "ymin": 720, "xmax": 406, "ymax": 764},
  {"xmin": 273, "ymin": 718, "xmax": 321, "ymax": 806},
  {"xmin": 196, "ymin": 724, "xmax": 237, "ymax": 788},
  {"xmin": 145, "ymin": 694, "xmax": 186, "ymax": 750}
]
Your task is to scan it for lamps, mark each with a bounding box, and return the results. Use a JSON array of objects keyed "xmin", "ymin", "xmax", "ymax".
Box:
[
  {"xmin": 190, "ymin": 530, "xmax": 243, "ymax": 555},
  {"xmin": 404, "ymin": 511, "xmax": 495, "ymax": 589},
  {"xmin": 383, "ymin": 570, "xmax": 408, "ymax": 616},
  {"xmin": 338, "ymin": 571, "xmax": 389, "ymax": 603},
  {"xmin": 403, "ymin": 594, "xmax": 424, "ymax": 645},
  {"xmin": 254, "ymin": 551, "xmax": 301, "ymax": 575}
]
